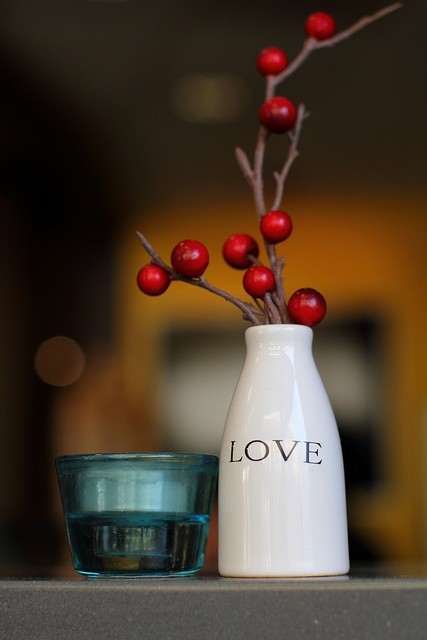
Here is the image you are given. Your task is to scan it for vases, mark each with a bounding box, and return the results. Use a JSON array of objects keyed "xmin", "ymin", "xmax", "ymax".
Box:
[{"xmin": 214, "ymin": 325, "xmax": 350, "ymax": 578}]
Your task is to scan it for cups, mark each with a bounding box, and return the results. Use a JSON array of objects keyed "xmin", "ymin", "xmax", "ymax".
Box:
[{"xmin": 55, "ymin": 452, "xmax": 219, "ymax": 580}]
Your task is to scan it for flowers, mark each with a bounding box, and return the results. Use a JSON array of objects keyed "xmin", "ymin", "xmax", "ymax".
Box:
[{"xmin": 132, "ymin": 1, "xmax": 427, "ymax": 323}]
